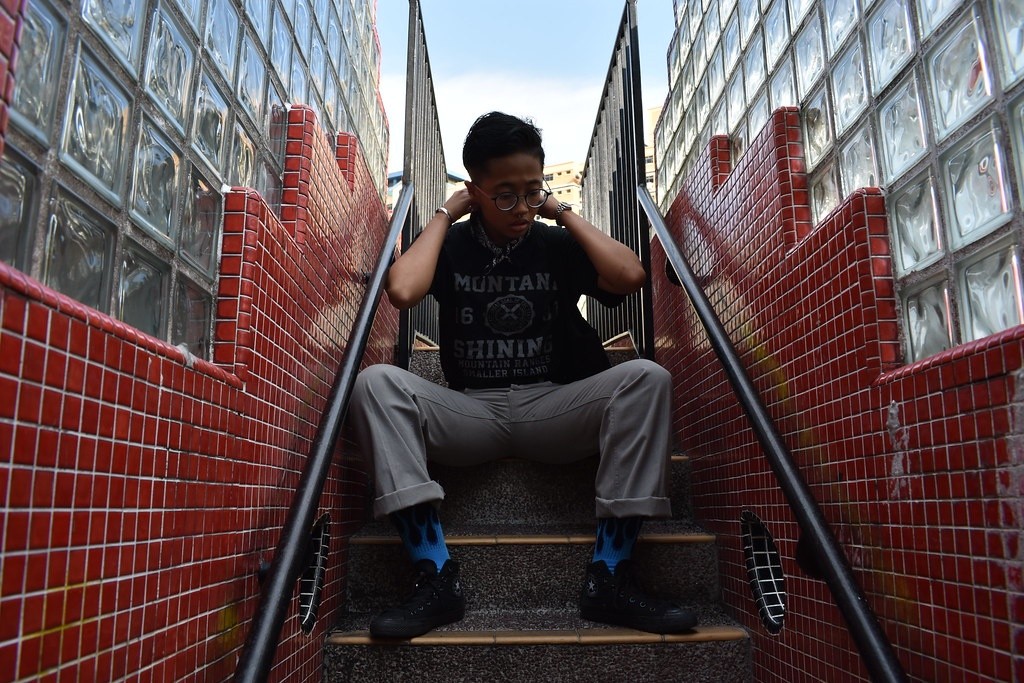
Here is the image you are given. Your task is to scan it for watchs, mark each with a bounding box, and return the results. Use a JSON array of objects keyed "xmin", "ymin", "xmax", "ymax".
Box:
[{"xmin": 555, "ymin": 203, "xmax": 572, "ymax": 226}]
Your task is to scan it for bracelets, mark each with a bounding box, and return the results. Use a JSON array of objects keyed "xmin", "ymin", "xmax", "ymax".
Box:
[{"xmin": 435, "ymin": 207, "xmax": 452, "ymax": 229}]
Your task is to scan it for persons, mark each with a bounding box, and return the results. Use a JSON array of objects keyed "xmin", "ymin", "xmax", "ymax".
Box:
[{"xmin": 349, "ymin": 110, "xmax": 695, "ymax": 641}]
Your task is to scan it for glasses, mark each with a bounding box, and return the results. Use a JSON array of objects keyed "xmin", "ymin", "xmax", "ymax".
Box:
[{"xmin": 470, "ymin": 177, "xmax": 553, "ymax": 212}]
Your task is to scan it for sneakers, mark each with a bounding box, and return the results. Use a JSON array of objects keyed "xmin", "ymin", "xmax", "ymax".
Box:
[
  {"xmin": 369, "ymin": 558, "xmax": 465, "ymax": 641},
  {"xmin": 577, "ymin": 559, "xmax": 698, "ymax": 634}
]
